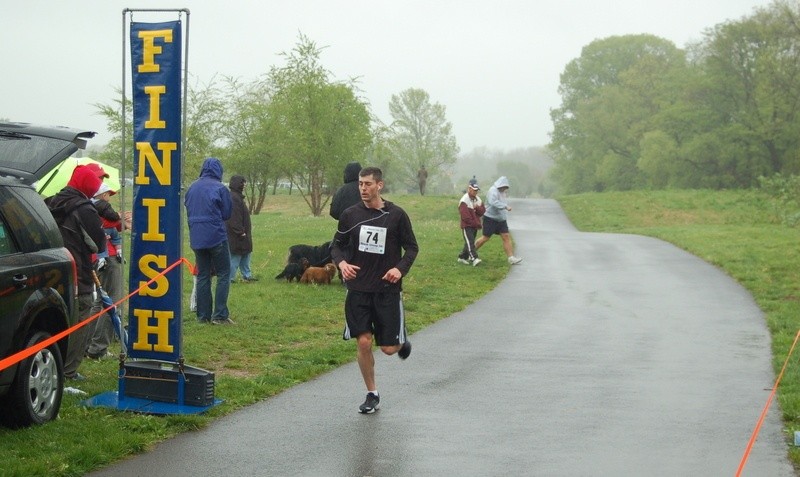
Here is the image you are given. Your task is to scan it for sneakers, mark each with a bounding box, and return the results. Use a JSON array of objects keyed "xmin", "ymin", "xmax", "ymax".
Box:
[
  {"xmin": 398, "ymin": 328, "xmax": 411, "ymax": 359},
  {"xmin": 358, "ymin": 392, "xmax": 380, "ymax": 413}
]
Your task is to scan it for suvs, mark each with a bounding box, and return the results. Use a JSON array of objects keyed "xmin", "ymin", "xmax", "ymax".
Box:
[{"xmin": 0, "ymin": 122, "xmax": 97, "ymax": 429}]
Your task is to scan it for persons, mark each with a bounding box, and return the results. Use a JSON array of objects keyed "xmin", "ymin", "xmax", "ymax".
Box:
[
  {"xmin": 417, "ymin": 165, "xmax": 427, "ymax": 195},
  {"xmin": 330, "ymin": 161, "xmax": 362, "ymax": 220},
  {"xmin": 475, "ymin": 176, "xmax": 522, "ymax": 264},
  {"xmin": 182, "ymin": 158, "xmax": 237, "ymax": 326},
  {"xmin": 332, "ymin": 167, "xmax": 420, "ymax": 414},
  {"xmin": 225, "ymin": 174, "xmax": 258, "ymax": 283},
  {"xmin": 469, "ymin": 176, "xmax": 477, "ymax": 184},
  {"xmin": 456, "ymin": 179, "xmax": 486, "ymax": 267},
  {"xmin": 44, "ymin": 164, "xmax": 130, "ymax": 380}
]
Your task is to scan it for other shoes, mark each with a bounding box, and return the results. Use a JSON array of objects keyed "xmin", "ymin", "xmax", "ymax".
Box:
[
  {"xmin": 65, "ymin": 373, "xmax": 85, "ymax": 381},
  {"xmin": 508, "ymin": 257, "xmax": 522, "ymax": 264},
  {"xmin": 213, "ymin": 318, "xmax": 234, "ymax": 325},
  {"xmin": 473, "ymin": 258, "xmax": 481, "ymax": 266},
  {"xmin": 198, "ymin": 319, "xmax": 210, "ymax": 324},
  {"xmin": 468, "ymin": 256, "xmax": 471, "ymax": 260},
  {"xmin": 98, "ymin": 260, "xmax": 107, "ymax": 271},
  {"xmin": 458, "ymin": 257, "xmax": 469, "ymax": 264},
  {"xmin": 244, "ymin": 277, "xmax": 258, "ymax": 281},
  {"xmin": 116, "ymin": 253, "xmax": 126, "ymax": 264},
  {"xmin": 230, "ymin": 279, "xmax": 238, "ymax": 283},
  {"xmin": 87, "ymin": 352, "xmax": 117, "ymax": 360}
]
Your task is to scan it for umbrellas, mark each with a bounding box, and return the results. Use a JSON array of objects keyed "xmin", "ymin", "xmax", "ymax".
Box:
[{"xmin": 37, "ymin": 157, "xmax": 119, "ymax": 197}]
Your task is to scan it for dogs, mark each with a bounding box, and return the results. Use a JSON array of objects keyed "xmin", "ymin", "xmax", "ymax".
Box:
[
  {"xmin": 276, "ymin": 239, "xmax": 333, "ymax": 283},
  {"xmin": 299, "ymin": 263, "xmax": 337, "ymax": 285}
]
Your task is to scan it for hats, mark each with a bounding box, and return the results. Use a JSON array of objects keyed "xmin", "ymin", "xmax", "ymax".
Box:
[
  {"xmin": 85, "ymin": 163, "xmax": 109, "ymax": 178},
  {"xmin": 93, "ymin": 183, "xmax": 116, "ymax": 197},
  {"xmin": 469, "ymin": 180, "xmax": 480, "ymax": 190}
]
